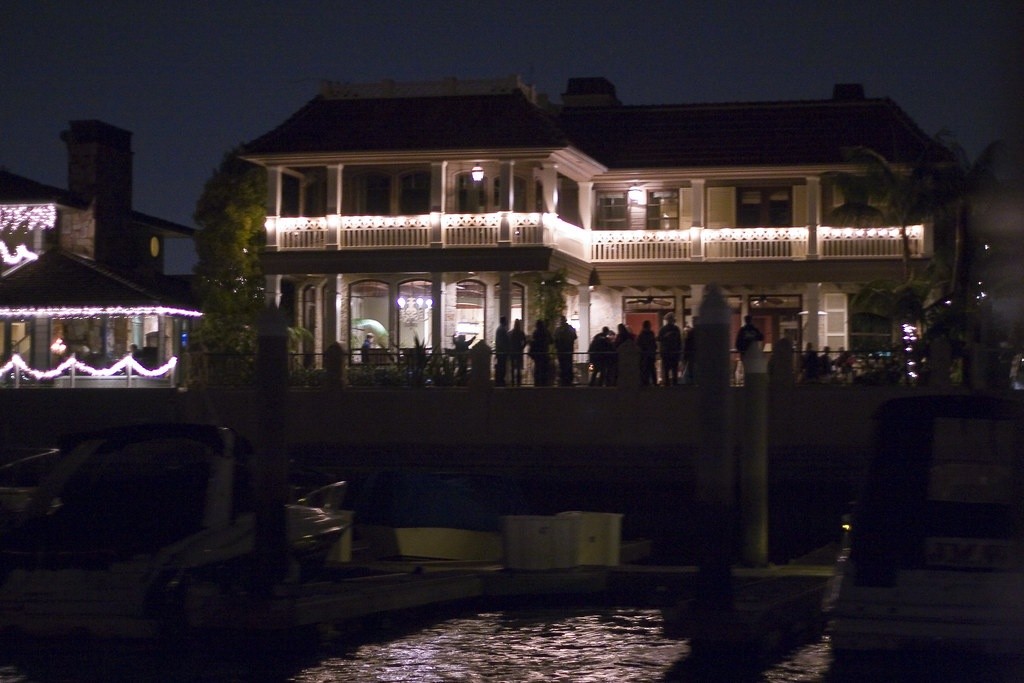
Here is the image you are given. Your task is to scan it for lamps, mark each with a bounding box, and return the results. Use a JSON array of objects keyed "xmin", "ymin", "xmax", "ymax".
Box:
[{"xmin": 472, "ymin": 162, "xmax": 485, "ymax": 181}]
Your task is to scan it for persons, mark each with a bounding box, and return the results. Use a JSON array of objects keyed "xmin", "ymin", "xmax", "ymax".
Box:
[
  {"xmin": 635, "ymin": 321, "xmax": 658, "ymax": 387},
  {"xmin": 131, "ymin": 342, "xmax": 143, "ymax": 358},
  {"xmin": 798, "ymin": 341, "xmax": 821, "ymax": 385},
  {"xmin": 492, "ymin": 316, "xmax": 509, "ymax": 387},
  {"xmin": 529, "ymin": 317, "xmax": 554, "ymax": 386},
  {"xmin": 615, "ymin": 323, "xmax": 634, "ymax": 348},
  {"xmin": 734, "ymin": 316, "xmax": 764, "ymax": 363},
  {"xmin": 361, "ymin": 333, "xmax": 375, "ymax": 366},
  {"xmin": 588, "ymin": 327, "xmax": 617, "ymax": 386},
  {"xmin": 837, "ymin": 348, "xmax": 853, "ymax": 376},
  {"xmin": 508, "ymin": 318, "xmax": 526, "ymax": 387},
  {"xmin": 453, "ymin": 332, "xmax": 477, "ymax": 381},
  {"xmin": 657, "ymin": 313, "xmax": 681, "ymax": 388},
  {"xmin": 820, "ymin": 346, "xmax": 834, "ymax": 380},
  {"xmin": 683, "ymin": 316, "xmax": 701, "ymax": 384},
  {"xmin": 553, "ymin": 317, "xmax": 577, "ymax": 384}
]
